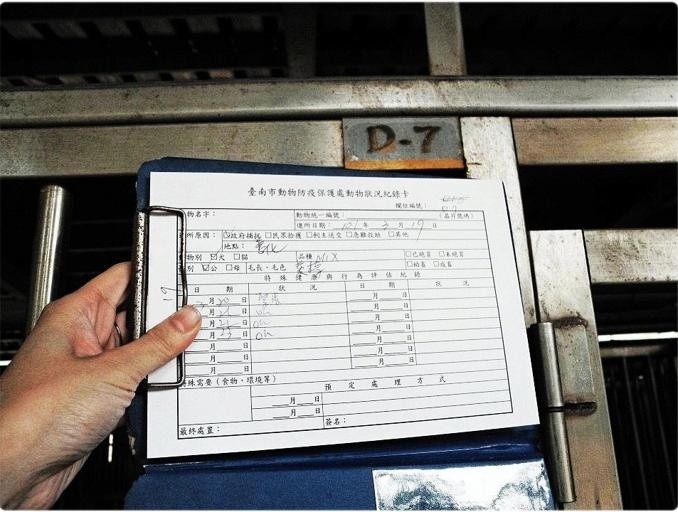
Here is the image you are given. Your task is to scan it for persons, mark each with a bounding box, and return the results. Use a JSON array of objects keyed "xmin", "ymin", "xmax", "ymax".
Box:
[{"xmin": 0, "ymin": 261, "xmax": 202, "ymax": 510}]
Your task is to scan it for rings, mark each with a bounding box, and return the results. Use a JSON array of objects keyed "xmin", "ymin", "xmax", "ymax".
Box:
[{"xmin": 113, "ymin": 321, "xmax": 123, "ymax": 345}]
